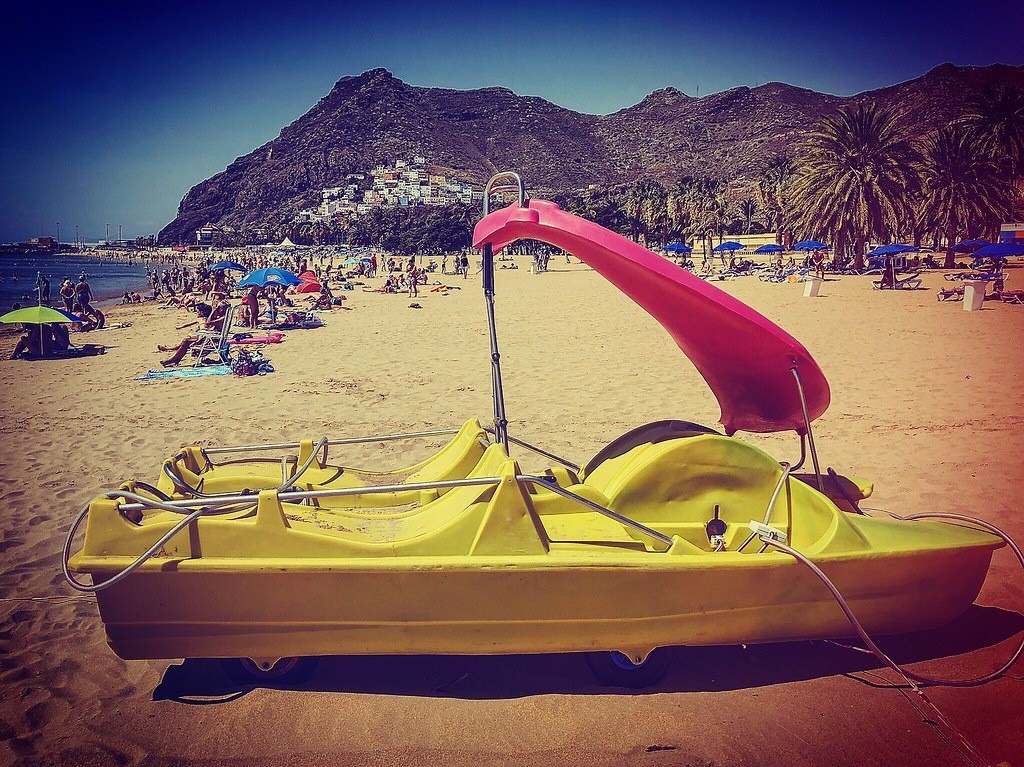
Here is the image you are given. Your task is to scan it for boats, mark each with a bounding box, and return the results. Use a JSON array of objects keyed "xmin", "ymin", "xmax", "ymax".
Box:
[{"xmin": 60, "ymin": 172, "xmax": 1006, "ymax": 673}]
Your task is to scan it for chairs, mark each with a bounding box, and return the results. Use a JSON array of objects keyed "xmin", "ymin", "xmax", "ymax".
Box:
[
  {"xmin": 157, "ymin": 264, "xmax": 398, "ymax": 369},
  {"xmin": 682, "ymin": 250, "xmax": 1024, "ymax": 305}
]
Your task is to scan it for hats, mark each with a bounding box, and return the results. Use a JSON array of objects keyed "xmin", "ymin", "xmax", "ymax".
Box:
[
  {"xmin": 63, "ymin": 280, "xmax": 72, "ymax": 286},
  {"xmin": 241, "ymin": 296, "xmax": 248, "ymax": 303},
  {"xmin": 78, "ymin": 276, "xmax": 86, "ymax": 279}
]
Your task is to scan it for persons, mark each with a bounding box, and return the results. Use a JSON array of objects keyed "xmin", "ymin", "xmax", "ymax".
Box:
[
  {"xmin": 651, "ymin": 246, "xmax": 1024, "ymax": 305},
  {"xmin": 0, "ymin": 241, "xmax": 584, "ymax": 367}
]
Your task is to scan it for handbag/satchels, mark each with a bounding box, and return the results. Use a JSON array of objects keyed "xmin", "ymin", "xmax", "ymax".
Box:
[
  {"xmin": 285, "ymin": 313, "xmax": 301, "ymax": 324},
  {"xmin": 277, "ymin": 299, "xmax": 283, "ymax": 306},
  {"xmin": 231, "ymin": 348, "xmax": 261, "ymax": 376},
  {"xmin": 288, "ymin": 290, "xmax": 295, "ymax": 295},
  {"xmin": 331, "ymin": 297, "xmax": 341, "ymax": 306}
]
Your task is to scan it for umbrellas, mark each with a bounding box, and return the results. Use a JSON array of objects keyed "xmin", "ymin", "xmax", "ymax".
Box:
[
  {"xmin": 344, "ymin": 257, "xmax": 360, "ymax": 274},
  {"xmin": 213, "ymin": 261, "xmax": 248, "ymax": 271},
  {"xmin": 866, "ymin": 243, "xmax": 920, "ymax": 289},
  {"xmin": 713, "ymin": 241, "xmax": 746, "ymax": 255},
  {"xmin": 359, "ymin": 257, "xmax": 372, "ymax": 263},
  {"xmin": 952, "ymin": 238, "xmax": 991, "ymax": 272},
  {"xmin": 662, "ymin": 243, "xmax": 692, "ymax": 253},
  {"xmin": 0, "ymin": 305, "xmax": 83, "ymax": 354},
  {"xmin": 788, "ymin": 238, "xmax": 832, "ymax": 275},
  {"xmin": 755, "ymin": 244, "xmax": 786, "ymax": 262},
  {"xmin": 968, "ymin": 242, "xmax": 1024, "ymax": 258},
  {"xmin": 237, "ymin": 266, "xmax": 302, "ymax": 322}
]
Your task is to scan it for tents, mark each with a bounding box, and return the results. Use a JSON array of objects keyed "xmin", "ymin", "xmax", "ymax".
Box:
[
  {"xmin": 277, "ymin": 237, "xmax": 297, "ymax": 250},
  {"xmin": 297, "ymin": 272, "xmax": 322, "ymax": 293}
]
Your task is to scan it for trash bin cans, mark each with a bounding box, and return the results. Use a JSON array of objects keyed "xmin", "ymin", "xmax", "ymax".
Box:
[
  {"xmin": 962, "ymin": 279, "xmax": 989, "ymax": 312},
  {"xmin": 803, "ymin": 276, "xmax": 825, "ymax": 298}
]
